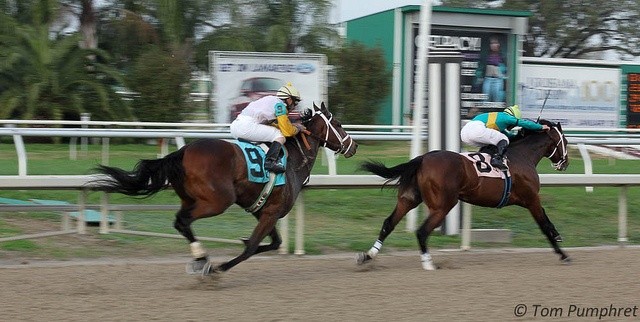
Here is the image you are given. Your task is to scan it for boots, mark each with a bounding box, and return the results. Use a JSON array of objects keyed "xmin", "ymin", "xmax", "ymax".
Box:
[
  {"xmin": 264, "ymin": 142, "xmax": 286, "ymax": 174},
  {"xmin": 491, "ymin": 140, "xmax": 510, "ymax": 170}
]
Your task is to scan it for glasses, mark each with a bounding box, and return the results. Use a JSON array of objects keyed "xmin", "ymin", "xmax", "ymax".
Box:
[{"xmin": 291, "ymin": 97, "xmax": 299, "ymax": 107}]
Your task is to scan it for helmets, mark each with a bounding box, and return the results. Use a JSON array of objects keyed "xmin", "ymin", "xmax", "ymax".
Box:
[
  {"xmin": 505, "ymin": 106, "xmax": 522, "ymax": 119},
  {"xmin": 277, "ymin": 86, "xmax": 301, "ymax": 100}
]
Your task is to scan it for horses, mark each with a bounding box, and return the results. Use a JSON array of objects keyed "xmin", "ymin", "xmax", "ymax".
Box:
[
  {"xmin": 80, "ymin": 101, "xmax": 359, "ymax": 277},
  {"xmin": 354, "ymin": 119, "xmax": 576, "ymax": 271}
]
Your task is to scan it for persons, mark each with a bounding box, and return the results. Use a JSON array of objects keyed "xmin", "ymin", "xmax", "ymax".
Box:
[
  {"xmin": 460, "ymin": 105, "xmax": 550, "ymax": 170},
  {"xmin": 478, "ymin": 37, "xmax": 508, "ymax": 101},
  {"xmin": 230, "ymin": 82, "xmax": 305, "ymax": 173}
]
